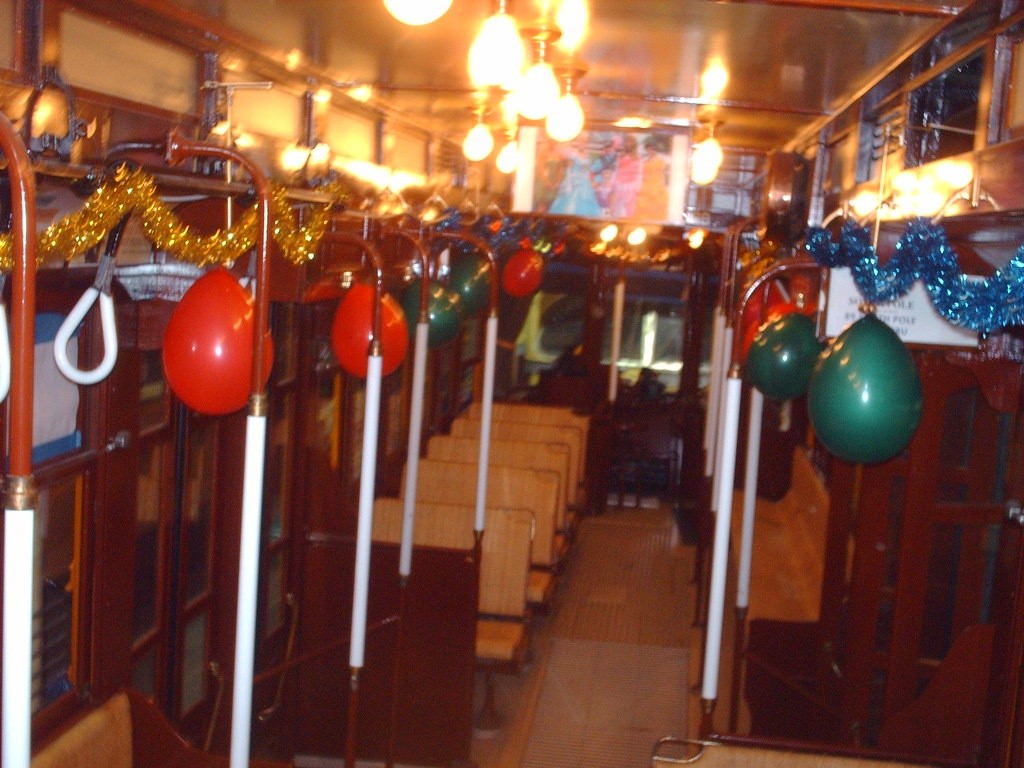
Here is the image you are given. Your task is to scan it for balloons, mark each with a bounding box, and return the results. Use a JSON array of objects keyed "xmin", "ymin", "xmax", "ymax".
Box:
[
  {"xmin": 162, "ymin": 264, "xmax": 274, "ymax": 415},
  {"xmin": 501, "ymin": 249, "xmax": 542, "ymax": 296},
  {"xmin": 331, "ymin": 255, "xmax": 490, "ymax": 377},
  {"xmin": 746, "ymin": 312, "xmax": 821, "ymax": 400},
  {"xmin": 807, "ymin": 314, "xmax": 922, "ymax": 463}
]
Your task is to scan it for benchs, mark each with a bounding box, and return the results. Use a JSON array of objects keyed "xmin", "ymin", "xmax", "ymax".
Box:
[
  {"xmin": 30, "ymin": 678, "xmax": 291, "ymax": 768},
  {"xmin": 718, "ymin": 445, "xmax": 854, "ymax": 679},
  {"xmin": 280, "ymin": 398, "xmax": 591, "ymax": 767}
]
